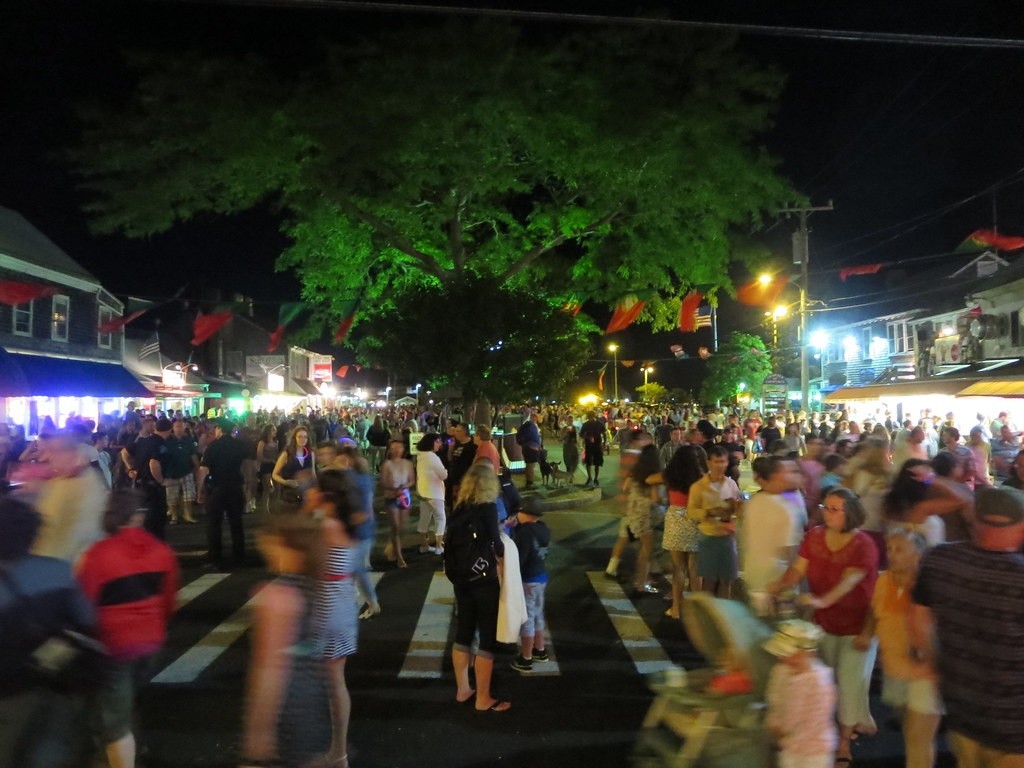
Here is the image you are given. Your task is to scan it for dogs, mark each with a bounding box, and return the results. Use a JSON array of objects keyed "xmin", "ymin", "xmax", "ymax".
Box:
[{"xmin": 537, "ymin": 449, "xmax": 576, "ymax": 493}]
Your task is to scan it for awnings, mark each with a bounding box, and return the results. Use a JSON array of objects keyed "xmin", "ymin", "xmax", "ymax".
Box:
[
  {"xmin": 821, "ymin": 385, "xmax": 896, "ymax": 403},
  {"xmin": 882, "ymin": 380, "xmax": 979, "ymax": 404},
  {"xmin": 955, "ymin": 377, "xmax": 1024, "ymax": 401},
  {"xmin": 0, "ymin": 348, "xmax": 156, "ymax": 398}
]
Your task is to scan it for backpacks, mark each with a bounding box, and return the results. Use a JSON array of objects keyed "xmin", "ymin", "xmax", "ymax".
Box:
[{"xmin": 444, "ymin": 502, "xmax": 496, "ymax": 584}]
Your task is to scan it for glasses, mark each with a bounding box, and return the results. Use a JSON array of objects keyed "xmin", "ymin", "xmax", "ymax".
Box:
[{"xmin": 818, "ymin": 504, "xmax": 845, "ymax": 513}]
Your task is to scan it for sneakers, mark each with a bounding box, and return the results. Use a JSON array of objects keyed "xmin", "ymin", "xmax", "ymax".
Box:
[
  {"xmin": 510, "ymin": 653, "xmax": 533, "ymax": 672},
  {"xmin": 532, "ymin": 646, "xmax": 549, "ymax": 662}
]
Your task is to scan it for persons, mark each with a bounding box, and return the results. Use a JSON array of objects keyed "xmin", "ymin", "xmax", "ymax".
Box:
[{"xmin": 0, "ymin": 398, "xmax": 1024, "ymax": 768}]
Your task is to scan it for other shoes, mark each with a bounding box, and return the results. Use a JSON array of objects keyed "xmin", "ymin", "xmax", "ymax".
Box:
[
  {"xmin": 169, "ymin": 514, "xmax": 179, "ymax": 525},
  {"xmin": 636, "ymin": 584, "xmax": 659, "ymax": 593},
  {"xmin": 594, "ymin": 479, "xmax": 599, "ymax": 486},
  {"xmin": 419, "ymin": 545, "xmax": 435, "ymax": 552},
  {"xmin": 586, "ymin": 478, "xmax": 592, "ymax": 485},
  {"xmin": 359, "ymin": 604, "xmax": 381, "ymax": 620},
  {"xmin": 385, "ymin": 544, "xmax": 396, "ymax": 562},
  {"xmin": 434, "ymin": 547, "xmax": 444, "ymax": 554},
  {"xmin": 397, "ymin": 559, "xmax": 408, "ymax": 568}
]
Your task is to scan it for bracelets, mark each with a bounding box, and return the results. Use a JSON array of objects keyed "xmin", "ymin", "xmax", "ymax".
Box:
[{"xmin": 128, "ymin": 468, "xmax": 136, "ymax": 474}]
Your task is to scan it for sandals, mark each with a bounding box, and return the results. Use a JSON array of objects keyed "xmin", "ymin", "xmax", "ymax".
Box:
[{"xmin": 301, "ymin": 753, "xmax": 348, "ymax": 768}]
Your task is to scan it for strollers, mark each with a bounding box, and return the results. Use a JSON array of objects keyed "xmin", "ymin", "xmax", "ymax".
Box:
[{"xmin": 636, "ymin": 592, "xmax": 813, "ymax": 768}]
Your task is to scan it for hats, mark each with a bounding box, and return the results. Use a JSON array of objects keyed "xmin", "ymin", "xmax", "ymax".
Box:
[
  {"xmin": 977, "ymin": 485, "xmax": 1024, "ymax": 527},
  {"xmin": 215, "ymin": 417, "xmax": 236, "ymax": 429},
  {"xmin": 518, "ymin": 497, "xmax": 543, "ymax": 516},
  {"xmin": 763, "ymin": 619, "xmax": 826, "ymax": 657}
]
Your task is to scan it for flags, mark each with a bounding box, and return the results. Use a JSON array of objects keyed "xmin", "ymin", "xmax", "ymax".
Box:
[
  {"xmin": 677, "ymin": 304, "xmax": 712, "ymax": 329},
  {"xmin": 137, "ymin": 332, "xmax": 160, "ymax": 362}
]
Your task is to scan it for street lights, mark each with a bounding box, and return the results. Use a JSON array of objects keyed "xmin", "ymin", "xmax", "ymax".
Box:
[
  {"xmin": 608, "ymin": 344, "xmax": 617, "ymax": 402},
  {"xmin": 760, "ymin": 274, "xmax": 808, "ymax": 412},
  {"xmin": 640, "ymin": 367, "xmax": 654, "ymax": 401},
  {"xmin": 329, "ymin": 382, "xmax": 423, "ymax": 406},
  {"xmin": 765, "ymin": 305, "xmax": 786, "ymax": 347}
]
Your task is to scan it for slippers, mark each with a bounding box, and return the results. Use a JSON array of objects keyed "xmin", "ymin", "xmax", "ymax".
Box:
[
  {"xmin": 474, "ymin": 700, "xmax": 512, "ymax": 713},
  {"xmin": 850, "ymin": 727, "xmax": 879, "ymax": 741},
  {"xmin": 455, "ymin": 686, "xmax": 475, "ymax": 703},
  {"xmin": 835, "ymin": 754, "xmax": 855, "ymax": 768}
]
